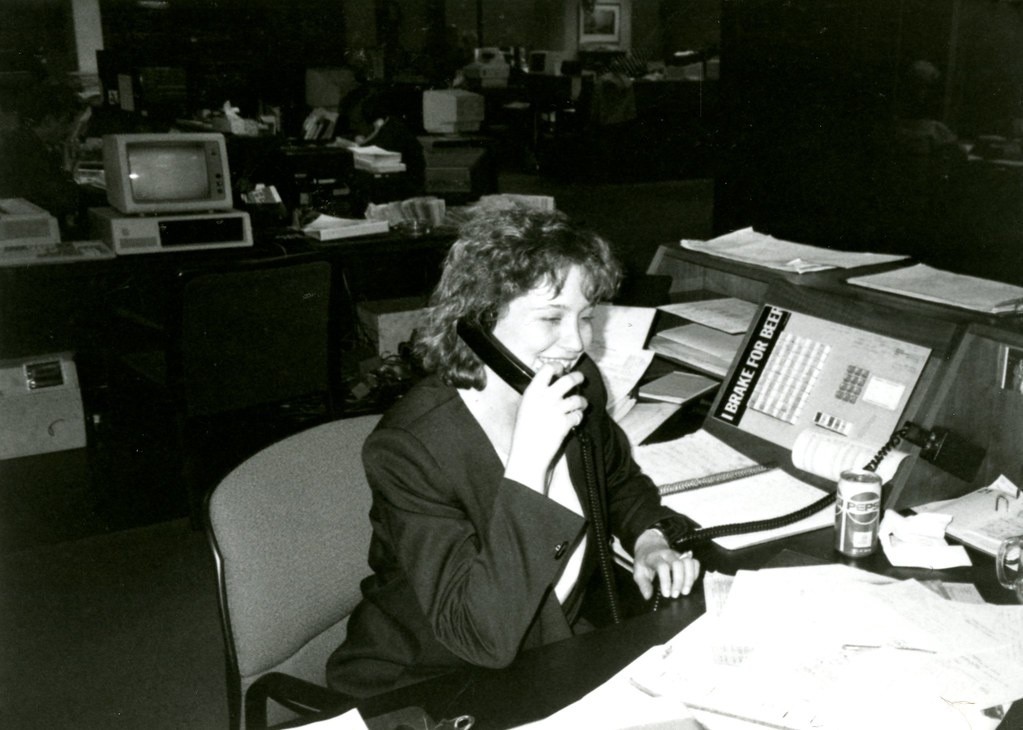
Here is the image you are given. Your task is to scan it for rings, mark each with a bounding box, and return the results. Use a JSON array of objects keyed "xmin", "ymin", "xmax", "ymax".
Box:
[{"xmin": 680, "ymin": 550, "xmax": 692, "ymax": 558}]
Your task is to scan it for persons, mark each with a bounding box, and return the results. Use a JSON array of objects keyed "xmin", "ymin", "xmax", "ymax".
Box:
[
  {"xmin": 326, "ymin": 204, "xmax": 699, "ymax": 698},
  {"xmin": 903, "ymin": 60, "xmax": 968, "ymax": 161},
  {"xmin": 0, "ymin": 82, "xmax": 108, "ymax": 238}
]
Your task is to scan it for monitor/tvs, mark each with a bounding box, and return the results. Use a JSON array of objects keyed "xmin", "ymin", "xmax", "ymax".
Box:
[
  {"xmin": 306, "ymin": 66, "xmax": 364, "ymax": 112},
  {"xmin": 102, "ymin": 132, "xmax": 232, "ymax": 216},
  {"xmin": 422, "ymin": 89, "xmax": 484, "ymax": 140},
  {"xmin": 530, "ymin": 50, "xmax": 555, "ymax": 76}
]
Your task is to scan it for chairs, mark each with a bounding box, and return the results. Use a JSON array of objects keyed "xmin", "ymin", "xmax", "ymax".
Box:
[{"xmin": 199, "ymin": 407, "xmax": 386, "ymax": 730}]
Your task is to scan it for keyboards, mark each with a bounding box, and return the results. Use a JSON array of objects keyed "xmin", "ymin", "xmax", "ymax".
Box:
[{"xmin": 0, "ymin": 239, "xmax": 116, "ymax": 267}]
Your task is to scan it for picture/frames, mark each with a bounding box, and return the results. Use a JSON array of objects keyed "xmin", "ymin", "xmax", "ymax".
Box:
[{"xmin": 577, "ymin": 3, "xmax": 622, "ymax": 46}]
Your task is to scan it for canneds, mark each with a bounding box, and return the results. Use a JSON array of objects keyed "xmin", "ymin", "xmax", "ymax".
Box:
[{"xmin": 833, "ymin": 468, "xmax": 882, "ymax": 557}]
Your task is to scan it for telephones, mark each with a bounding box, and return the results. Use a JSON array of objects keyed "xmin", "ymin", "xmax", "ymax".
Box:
[{"xmin": 456, "ymin": 304, "xmax": 579, "ymax": 435}]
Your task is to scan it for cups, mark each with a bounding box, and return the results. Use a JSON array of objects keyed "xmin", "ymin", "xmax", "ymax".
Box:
[{"xmin": 995, "ymin": 491, "xmax": 1023, "ymax": 602}]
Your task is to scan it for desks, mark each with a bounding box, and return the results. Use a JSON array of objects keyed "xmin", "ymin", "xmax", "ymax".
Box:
[
  {"xmin": 262, "ymin": 238, "xmax": 1023, "ymax": 730},
  {"xmin": 0, "ymin": 227, "xmax": 455, "ymax": 553}
]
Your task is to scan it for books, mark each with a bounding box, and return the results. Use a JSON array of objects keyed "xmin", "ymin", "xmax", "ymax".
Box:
[
  {"xmin": 638, "ymin": 370, "xmax": 718, "ymax": 405},
  {"xmin": 304, "ymin": 107, "xmax": 407, "ymax": 173},
  {"xmin": 632, "ymin": 428, "xmax": 836, "ymax": 552},
  {"xmin": 910, "ymin": 474, "xmax": 1023, "ymax": 574}
]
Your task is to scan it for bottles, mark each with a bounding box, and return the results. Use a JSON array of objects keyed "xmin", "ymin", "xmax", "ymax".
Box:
[{"xmin": 293, "ymin": 172, "xmax": 313, "ymax": 212}]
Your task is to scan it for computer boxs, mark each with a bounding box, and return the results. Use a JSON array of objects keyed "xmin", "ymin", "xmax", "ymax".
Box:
[{"xmin": 90, "ymin": 207, "xmax": 254, "ymax": 256}]
